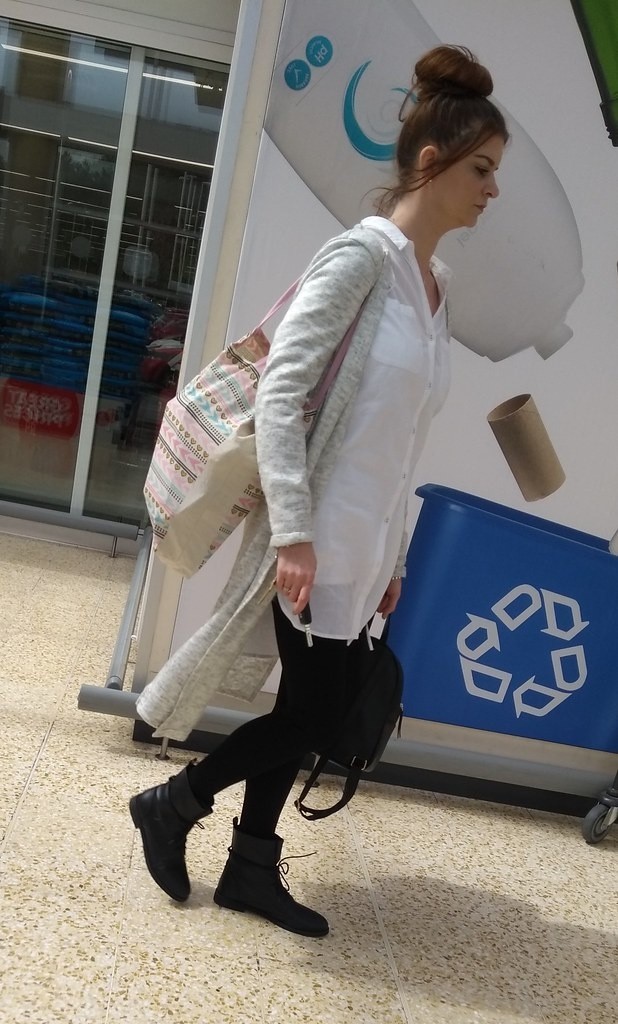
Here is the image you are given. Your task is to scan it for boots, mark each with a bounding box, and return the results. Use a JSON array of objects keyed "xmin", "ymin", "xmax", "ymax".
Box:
[
  {"xmin": 213, "ymin": 817, "xmax": 330, "ymax": 936},
  {"xmin": 129, "ymin": 763, "xmax": 214, "ymax": 902}
]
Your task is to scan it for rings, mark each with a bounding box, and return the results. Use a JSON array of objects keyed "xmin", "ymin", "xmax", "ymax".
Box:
[{"xmin": 283, "ymin": 587, "xmax": 291, "ymax": 592}]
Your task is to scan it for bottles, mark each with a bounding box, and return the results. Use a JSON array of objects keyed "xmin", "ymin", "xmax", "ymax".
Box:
[
  {"xmin": 568, "ymin": 0, "xmax": 618, "ymax": 147},
  {"xmin": 264, "ymin": 0, "xmax": 585, "ymax": 363}
]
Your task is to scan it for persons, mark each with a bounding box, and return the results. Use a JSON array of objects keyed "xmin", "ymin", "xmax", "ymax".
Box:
[{"xmin": 129, "ymin": 47, "xmax": 507, "ymax": 938}]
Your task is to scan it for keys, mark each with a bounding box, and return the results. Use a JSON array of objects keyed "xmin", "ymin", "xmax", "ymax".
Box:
[
  {"xmin": 298, "ymin": 602, "xmax": 313, "ymax": 647},
  {"xmin": 257, "ymin": 576, "xmax": 276, "ymax": 604}
]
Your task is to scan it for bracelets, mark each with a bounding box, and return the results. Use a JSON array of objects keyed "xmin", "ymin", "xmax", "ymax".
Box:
[{"xmin": 391, "ymin": 576, "xmax": 401, "ymax": 580}]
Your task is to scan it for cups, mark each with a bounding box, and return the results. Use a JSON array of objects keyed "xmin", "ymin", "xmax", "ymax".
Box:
[{"xmin": 485, "ymin": 394, "xmax": 566, "ymax": 501}]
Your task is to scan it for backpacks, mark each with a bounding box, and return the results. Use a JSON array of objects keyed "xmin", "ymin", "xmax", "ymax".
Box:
[{"xmin": 293, "ymin": 612, "xmax": 404, "ymax": 821}]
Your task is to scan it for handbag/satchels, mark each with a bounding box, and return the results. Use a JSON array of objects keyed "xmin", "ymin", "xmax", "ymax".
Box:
[{"xmin": 144, "ymin": 261, "xmax": 367, "ymax": 578}]
